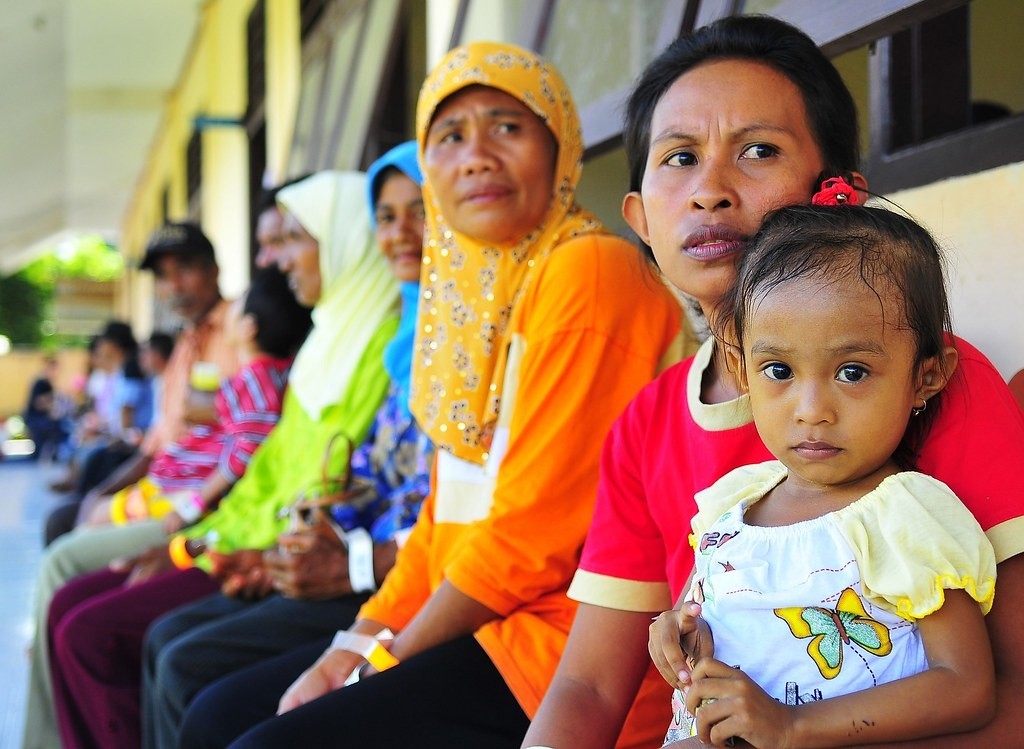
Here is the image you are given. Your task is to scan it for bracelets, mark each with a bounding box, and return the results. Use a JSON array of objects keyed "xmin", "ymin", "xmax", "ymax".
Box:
[
  {"xmin": 332, "ymin": 630, "xmax": 372, "ymax": 655},
  {"xmin": 178, "ymin": 499, "xmax": 203, "ymax": 525},
  {"xmin": 344, "ymin": 663, "xmax": 367, "ymax": 686},
  {"xmin": 348, "ymin": 527, "xmax": 376, "ymax": 594},
  {"xmin": 364, "ymin": 638, "xmax": 399, "ymax": 673}
]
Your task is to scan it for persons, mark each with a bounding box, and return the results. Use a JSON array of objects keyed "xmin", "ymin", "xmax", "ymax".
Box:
[
  {"xmin": 648, "ymin": 165, "xmax": 998, "ymax": 749},
  {"xmin": 518, "ymin": 11, "xmax": 1024, "ymax": 749},
  {"xmin": 23, "ymin": 41, "xmax": 702, "ymax": 749}
]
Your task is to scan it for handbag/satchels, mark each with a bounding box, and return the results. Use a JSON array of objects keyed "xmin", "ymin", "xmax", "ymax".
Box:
[{"xmin": 279, "ymin": 430, "xmax": 385, "ymax": 562}]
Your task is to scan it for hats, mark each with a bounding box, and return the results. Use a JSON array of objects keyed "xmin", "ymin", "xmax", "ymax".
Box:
[{"xmin": 138, "ymin": 223, "xmax": 216, "ymax": 272}]
